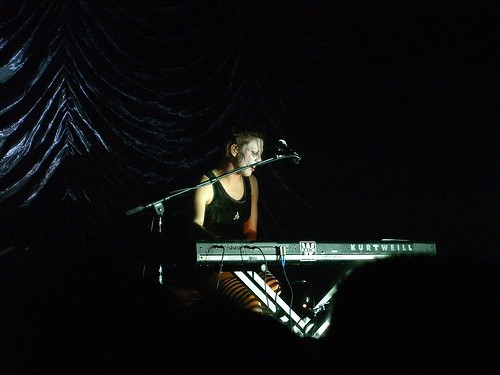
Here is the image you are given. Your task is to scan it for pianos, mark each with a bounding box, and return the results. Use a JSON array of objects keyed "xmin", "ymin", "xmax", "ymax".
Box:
[{"xmin": 179, "ymin": 230, "xmax": 437, "ymax": 271}]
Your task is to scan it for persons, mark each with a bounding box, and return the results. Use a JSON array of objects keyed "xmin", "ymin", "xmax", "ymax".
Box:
[{"xmin": 189, "ymin": 130, "xmax": 284, "ymax": 315}]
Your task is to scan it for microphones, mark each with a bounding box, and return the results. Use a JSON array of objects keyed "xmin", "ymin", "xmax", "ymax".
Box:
[{"xmin": 273, "ymin": 138, "xmax": 301, "ymax": 162}]
